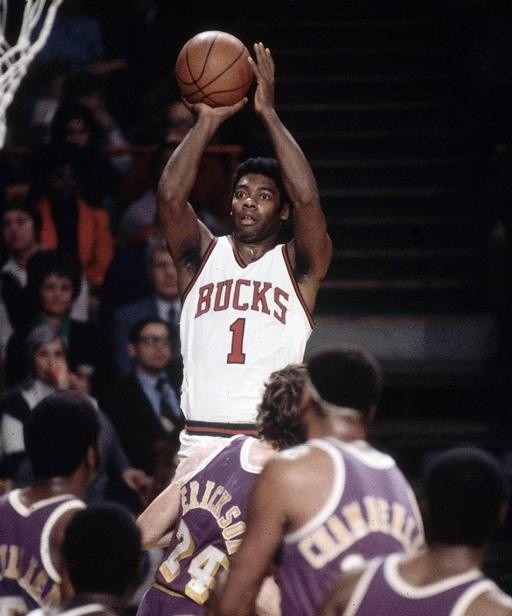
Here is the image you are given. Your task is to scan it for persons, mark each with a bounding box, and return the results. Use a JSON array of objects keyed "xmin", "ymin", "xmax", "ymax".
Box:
[{"xmin": 0, "ymin": 1, "xmax": 511, "ymax": 615}]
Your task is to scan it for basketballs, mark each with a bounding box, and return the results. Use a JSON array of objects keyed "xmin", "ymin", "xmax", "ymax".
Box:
[{"xmin": 176, "ymin": 31, "xmax": 253, "ymax": 104}]
